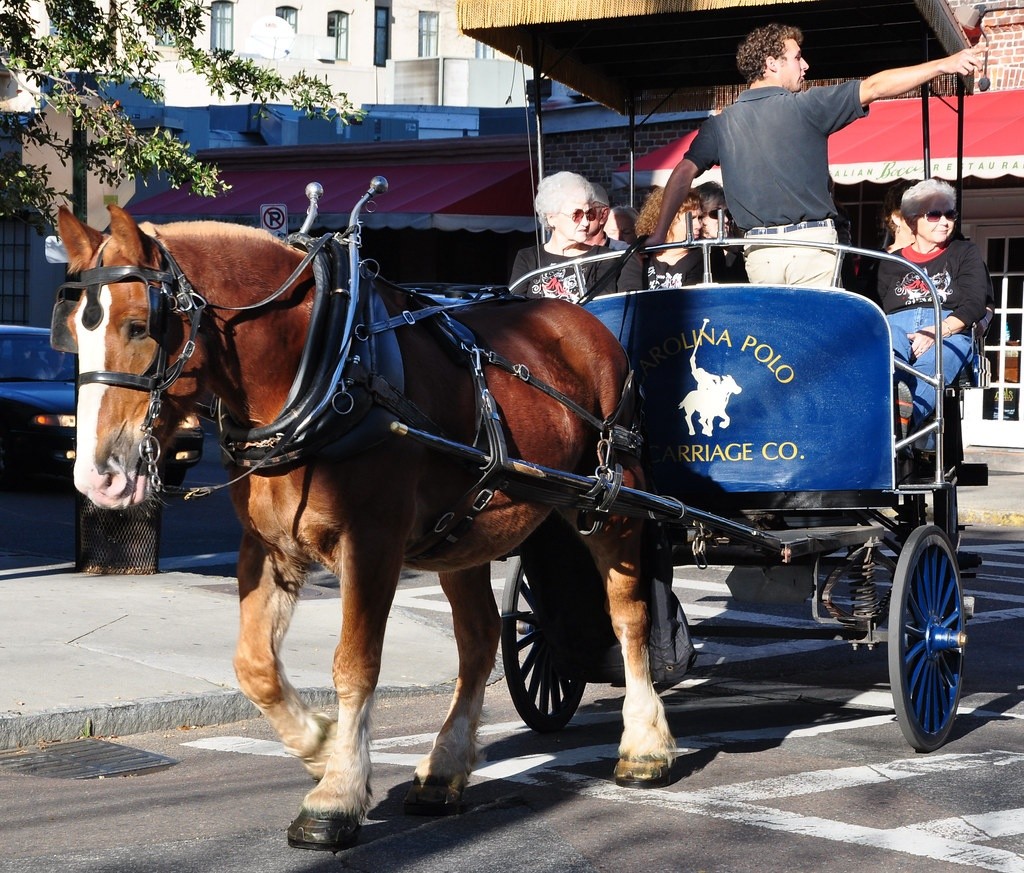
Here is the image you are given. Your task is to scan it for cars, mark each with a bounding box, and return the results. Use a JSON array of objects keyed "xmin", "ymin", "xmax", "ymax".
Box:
[{"xmin": 0, "ymin": 324, "xmax": 204, "ymax": 496}]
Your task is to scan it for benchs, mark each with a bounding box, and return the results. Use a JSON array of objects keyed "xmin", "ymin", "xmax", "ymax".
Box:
[{"xmin": 507, "ymin": 237, "xmax": 996, "ymax": 511}]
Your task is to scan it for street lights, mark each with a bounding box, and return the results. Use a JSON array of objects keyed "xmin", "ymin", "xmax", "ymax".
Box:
[{"xmin": 525, "ymin": 77, "xmax": 551, "ymax": 191}]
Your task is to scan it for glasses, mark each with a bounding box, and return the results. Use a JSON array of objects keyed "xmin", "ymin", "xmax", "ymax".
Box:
[
  {"xmin": 703, "ymin": 207, "xmax": 733, "ymax": 220},
  {"xmin": 558, "ymin": 206, "xmax": 598, "ymax": 224},
  {"xmin": 925, "ymin": 209, "xmax": 959, "ymax": 221}
]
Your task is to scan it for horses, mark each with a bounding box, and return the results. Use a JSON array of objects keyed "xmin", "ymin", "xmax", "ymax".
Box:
[{"xmin": 52, "ymin": 204, "xmax": 676, "ymax": 853}]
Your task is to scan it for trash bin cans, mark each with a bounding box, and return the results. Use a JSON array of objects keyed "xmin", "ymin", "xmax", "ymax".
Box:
[{"xmin": 72, "ymin": 491, "xmax": 159, "ymax": 576}]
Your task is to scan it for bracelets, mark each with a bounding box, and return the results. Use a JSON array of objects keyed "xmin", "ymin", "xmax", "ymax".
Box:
[{"xmin": 943, "ymin": 320, "xmax": 952, "ymax": 336}]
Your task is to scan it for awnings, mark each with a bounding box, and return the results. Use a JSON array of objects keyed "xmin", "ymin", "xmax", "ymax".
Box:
[
  {"xmin": 612, "ymin": 89, "xmax": 1024, "ymax": 190},
  {"xmin": 123, "ymin": 152, "xmax": 542, "ymax": 233}
]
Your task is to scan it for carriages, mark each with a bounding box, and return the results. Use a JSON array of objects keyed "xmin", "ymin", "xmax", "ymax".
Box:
[{"xmin": 50, "ymin": 1, "xmax": 988, "ymax": 851}]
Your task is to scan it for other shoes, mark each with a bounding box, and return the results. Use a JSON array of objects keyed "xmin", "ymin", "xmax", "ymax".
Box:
[
  {"xmin": 893, "ymin": 381, "xmax": 914, "ymax": 442},
  {"xmin": 897, "ymin": 442, "xmax": 914, "ymax": 460}
]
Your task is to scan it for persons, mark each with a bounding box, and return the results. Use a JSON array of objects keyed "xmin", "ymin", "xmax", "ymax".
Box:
[
  {"xmin": 509, "ymin": 171, "xmax": 642, "ymax": 297},
  {"xmin": 603, "ymin": 204, "xmax": 641, "ymax": 243},
  {"xmin": 635, "ymin": 25, "xmax": 989, "ymax": 287},
  {"xmin": 617, "ymin": 181, "xmax": 750, "ymax": 294},
  {"xmin": 588, "ymin": 181, "xmax": 633, "ymax": 251},
  {"xmin": 832, "ymin": 176, "xmax": 986, "ymax": 437}
]
